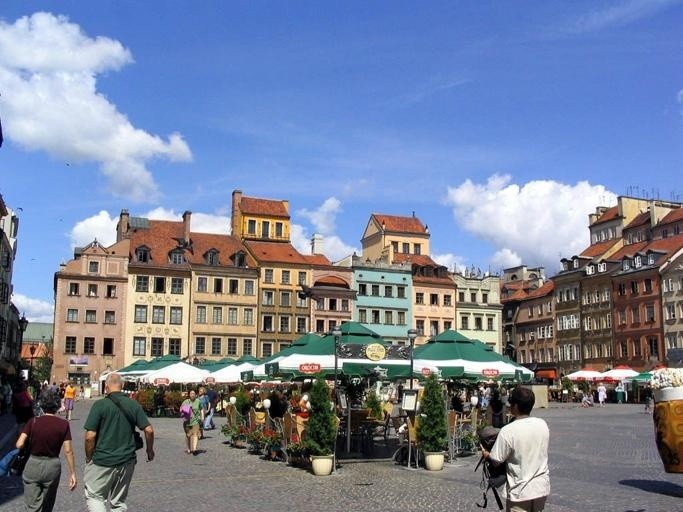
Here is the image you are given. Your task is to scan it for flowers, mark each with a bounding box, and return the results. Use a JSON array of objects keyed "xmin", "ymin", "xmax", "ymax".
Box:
[
  {"xmin": 117, "ymin": 380, "xmax": 221, "ymax": 417},
  {"xmin": 221, "ymin": 380, "xmax": 482, "ymax": 467}
]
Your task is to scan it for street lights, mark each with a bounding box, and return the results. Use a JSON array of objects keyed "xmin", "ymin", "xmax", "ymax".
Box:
[
  {"xmin": 406, "ymin": 329, "xmax": 419, "ymax": 390},
  {"xmin": 29, "ymin": 344, "xmax": 38, "ymax": 368}
]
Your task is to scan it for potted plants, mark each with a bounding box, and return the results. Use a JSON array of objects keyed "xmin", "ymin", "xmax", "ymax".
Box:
[
  {"xmin": 421, "ymin": 370, "xmax": 451, "ymax": 470},
  {"xmin": 304, "ymin": 372, "xmax": 340, "ymax": 476}
]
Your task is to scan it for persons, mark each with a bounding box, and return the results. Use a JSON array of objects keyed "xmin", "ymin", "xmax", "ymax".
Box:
[
  {"xmin": 597, "ymin": 383, "xmax": 607, "ymax": 407},
  {"xmin": 478, "ymin": 387, "xmax": 550, "ymax": 512},
  {"xmin": 16, "ymin": 389, "xmax": 77, "ymax": 512},
  {"xmin": 615, "ymin": 383, "xmax": 624, "ymax": 404},
  {"xmin": 0, "ymin": 379, "xmax": 84, "ymax": 435},
  {"xmin": 83, "ymin": 372, "xmax": 219, "ymax": 512},
  {"xmin": 581, "ymin": 392, "xmax": 594, "ymax": 408}
]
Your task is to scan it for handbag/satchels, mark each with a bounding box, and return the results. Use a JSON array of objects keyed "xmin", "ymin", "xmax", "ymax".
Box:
[
  {"xmin": 133, "ymin": 430, "xmax": 143, "ymax": 450},
  {"xmin": 0, "ymin": 448, "xmax": 29, "ymax": 481}
]
